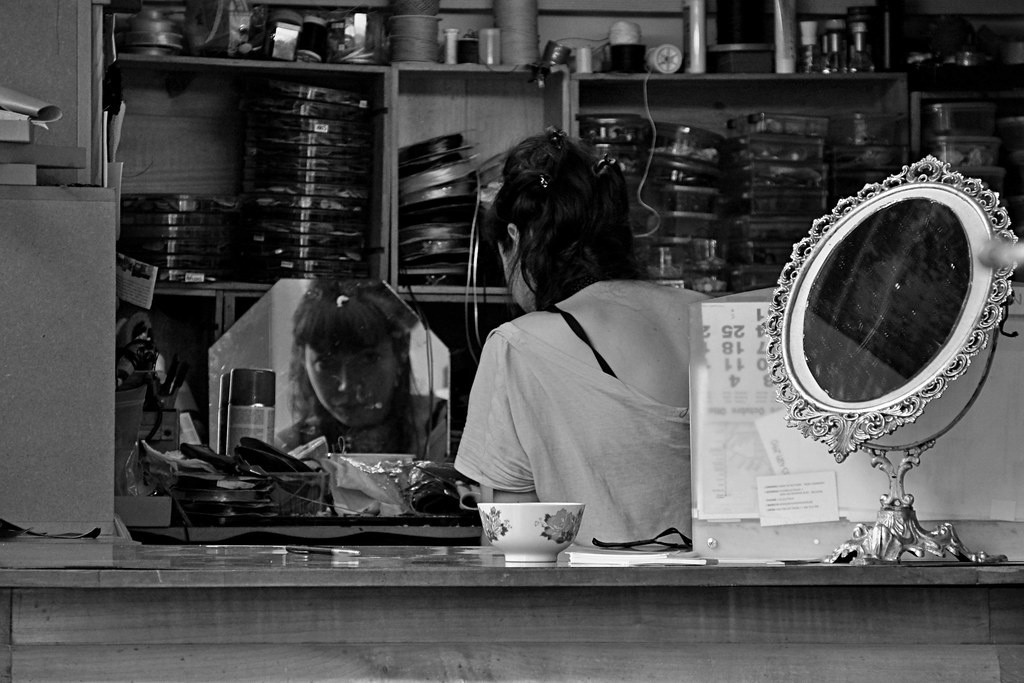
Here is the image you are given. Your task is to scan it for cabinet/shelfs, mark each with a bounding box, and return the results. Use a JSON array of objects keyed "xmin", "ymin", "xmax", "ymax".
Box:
[{"xmin": 75, "ymin": 0, "xmax": 1024, "ymax": 543}]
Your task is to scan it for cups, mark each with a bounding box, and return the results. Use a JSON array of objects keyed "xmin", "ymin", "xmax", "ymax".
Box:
[
  {"xmin": 543, "ymin": 41, "xmax": 570, "ymax": 65},
  {"xmin": 477, "ymin": 28, "xmax": 502, "ymax": 67},
  {"xmin": 575, "ymin": 48, "xmax": 593, "ymax": 74}
]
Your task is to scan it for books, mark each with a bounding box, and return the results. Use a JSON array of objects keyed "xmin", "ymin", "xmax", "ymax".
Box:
[{"xmin": 564, "ymin": 547, "xmax": 720, "ymax": 567}]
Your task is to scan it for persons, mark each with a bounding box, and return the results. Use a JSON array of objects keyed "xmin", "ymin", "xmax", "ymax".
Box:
[
  {"xmin": 451, "ymin": 127, "xmax": 706, "ymax": 550},
  {"xmin": 274, "ymin": 279, "xmax": 448, "ymax": 464}
]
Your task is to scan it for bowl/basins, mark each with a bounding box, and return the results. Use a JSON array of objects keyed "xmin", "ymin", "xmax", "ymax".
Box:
[
  {"xmin": 492, "ymin": 0, "xmax": 541, "ymax": 66},
  {"xmin": 477, "ymin": 502, "xmax": 586, "ymax": 563},
  {"xmin": 385, "ymin": 0, "xmax": 443, "ymax": 62},
  {"xmin": 328, "ymin": 453, "xmax": 418, "ymax": 516}
]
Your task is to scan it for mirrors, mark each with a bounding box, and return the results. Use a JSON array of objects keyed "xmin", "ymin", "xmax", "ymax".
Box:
[
  {"xmin": 209, "ymin": 278, "xmax": 451, "ymax": 460},
  {"xmin": 766, "ymin": 155, "xmax": 1021, "ymax": 564}
]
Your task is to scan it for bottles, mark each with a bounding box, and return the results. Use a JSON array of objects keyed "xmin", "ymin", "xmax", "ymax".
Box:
[{"xmin": 797, "ymin": 6, "xmax": 875, "ymax": 73}]
